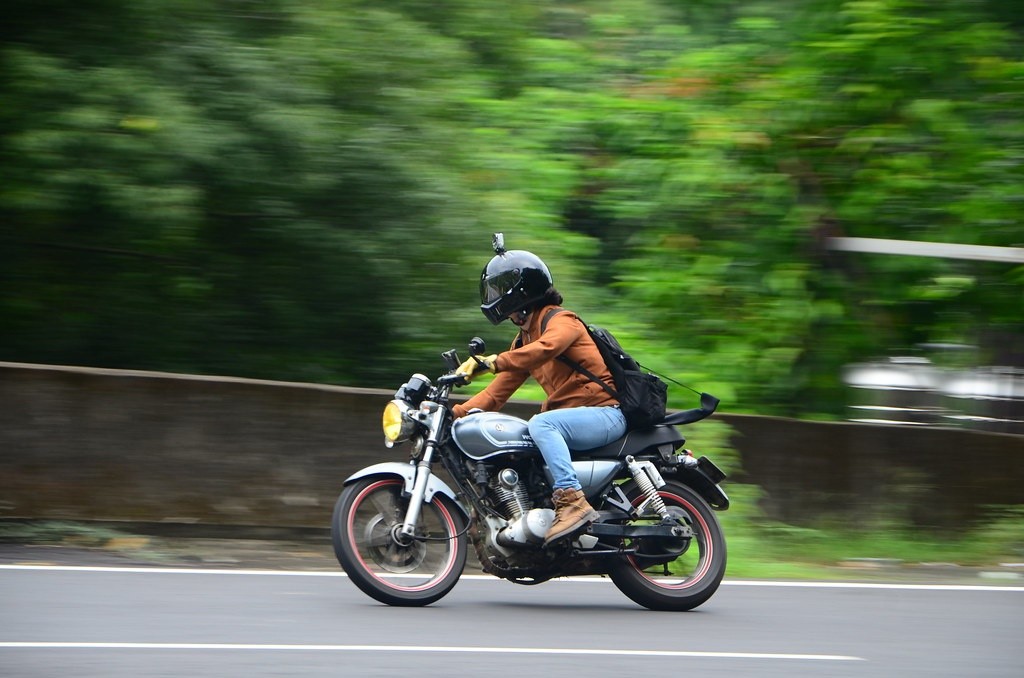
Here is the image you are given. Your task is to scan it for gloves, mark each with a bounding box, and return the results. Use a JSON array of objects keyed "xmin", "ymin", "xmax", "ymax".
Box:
[{"xmin": 455, "ymin": 353, "xmax": 497, "ymax": 381}]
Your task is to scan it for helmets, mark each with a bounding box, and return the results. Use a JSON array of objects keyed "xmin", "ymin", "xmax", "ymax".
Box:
[{"xmin": 480, "ymin": 250, "xmax": 553, "ymax": 325}]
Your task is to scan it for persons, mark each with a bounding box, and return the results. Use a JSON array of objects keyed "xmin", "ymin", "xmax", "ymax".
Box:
[{"xmin": 450, "ymin": 250, "xmax": 627, "ymax": 550}]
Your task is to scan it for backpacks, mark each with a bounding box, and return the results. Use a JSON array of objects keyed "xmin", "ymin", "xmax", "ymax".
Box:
[{"xmin": 515, "ymin": 307, "xmax": 668, "ymax": 430}]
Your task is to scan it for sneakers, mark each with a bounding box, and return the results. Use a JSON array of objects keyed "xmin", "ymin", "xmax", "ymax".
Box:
[{"xmin": 545, "ymin": 488, "xmax": 600, "ymax": 544}]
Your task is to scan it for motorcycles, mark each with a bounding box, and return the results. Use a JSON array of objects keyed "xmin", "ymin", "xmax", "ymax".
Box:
[{"xmin": 329, "ymin": 335, "xmax": 732, "ymax": 612}]
